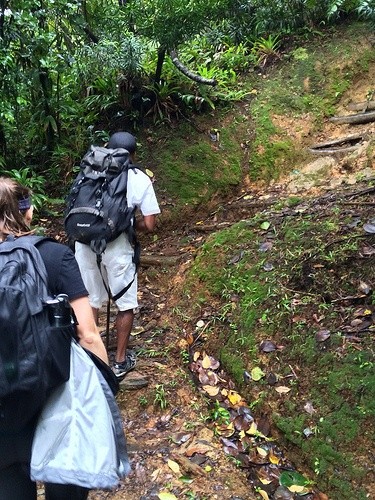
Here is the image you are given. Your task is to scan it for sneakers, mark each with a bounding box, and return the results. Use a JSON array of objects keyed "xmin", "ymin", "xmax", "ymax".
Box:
[{"xmin": 112, "ymin": 353, "xmax": 137, "ymax": 381}]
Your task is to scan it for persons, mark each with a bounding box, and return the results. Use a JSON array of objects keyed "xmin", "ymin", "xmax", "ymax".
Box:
[
  {"xmin": 0, "ymin": 177, "xmax": 109, "ymax": 500},
  {"xmin": 64, "ymin": 132, "xmax": 162, "ymax": 378}
]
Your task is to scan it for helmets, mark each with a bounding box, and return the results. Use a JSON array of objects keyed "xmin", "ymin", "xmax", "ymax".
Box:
[{"xmin": 108, "ymin": 132, "xmax": 137, "ymax": 153}]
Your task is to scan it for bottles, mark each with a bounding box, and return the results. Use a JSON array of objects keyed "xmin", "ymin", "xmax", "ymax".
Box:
[{"xmin": 51, "ymin": 294, "xmax": 71, "ymax": 327}]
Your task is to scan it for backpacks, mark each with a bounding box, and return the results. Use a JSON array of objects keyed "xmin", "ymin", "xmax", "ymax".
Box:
[
  {"xmin": 63, "ymin": 145, "xmax": 148, "ymax": 255},
  {"xmin": 0, "ymin": 232, "xmax": 82, "ymax": 404}
]
